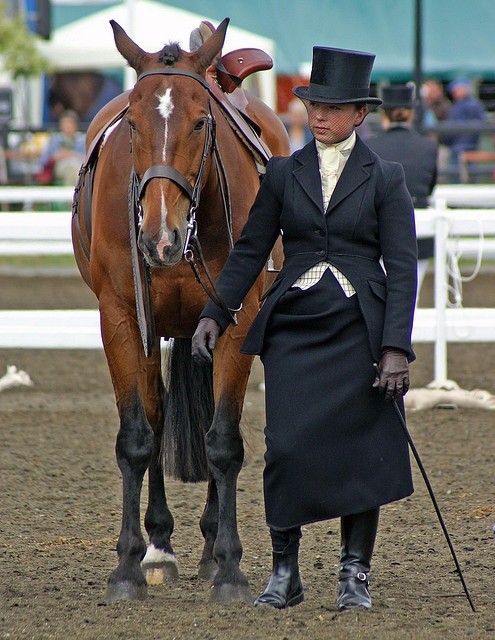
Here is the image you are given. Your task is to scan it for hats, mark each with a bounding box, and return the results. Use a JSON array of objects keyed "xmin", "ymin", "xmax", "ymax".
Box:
[
  {"xmin": 293, "ymin": 46, "xmax": 382, "ymax": 104},
  {"xmin": 380, "ymin": 87, "xmax": 414, "ymax": 109}
]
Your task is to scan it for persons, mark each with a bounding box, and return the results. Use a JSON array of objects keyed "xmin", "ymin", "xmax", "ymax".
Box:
[
  {"xmin": 364, "ymin": 81, "xmax": 438, "ymax": 255},
  {"xmin": 421, "ymin": 79, "xmax": 454, "ymax": 146},
  {"xmin": 189, "ymin": 44, "xmax": 419, "ymax": 612},
  {"xmin": 37, "ymin": 109, "xmax": 90, "ymax": 183},
  {"xmin": 440, "ymin": 73, "xmax": 487, "ymax": 183},
  {"xmin": 278, "ymin": 95, "xmax": 311, "ymax": 155}
]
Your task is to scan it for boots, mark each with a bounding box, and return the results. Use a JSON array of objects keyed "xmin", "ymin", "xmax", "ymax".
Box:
[
  {"xmin": 338, "ymin": 506, "xmax": 379, "ymax": 610},
  {"xmin": 254, "ymin": 550, "xmax": 304, "ymax": 608}
]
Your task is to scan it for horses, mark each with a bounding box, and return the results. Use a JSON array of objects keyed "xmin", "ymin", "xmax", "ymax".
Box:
[{"xmin": 70, "ymin": 16, "xmax": 292, "ymax": 609}]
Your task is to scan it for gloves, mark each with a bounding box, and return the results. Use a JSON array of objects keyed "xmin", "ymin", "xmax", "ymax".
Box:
[
  {"xmin": 191, "ymin": 317, "xmax": 220, "ymax": 366},
  {"xmin": 372, "ymin": 349, "xmax": 410, "ymax": 402}
]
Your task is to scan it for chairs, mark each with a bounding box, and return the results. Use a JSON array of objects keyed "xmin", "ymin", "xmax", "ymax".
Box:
[{"xmin": 459, "ymin": 120, "xmax": 495, "ymax": 181}]
